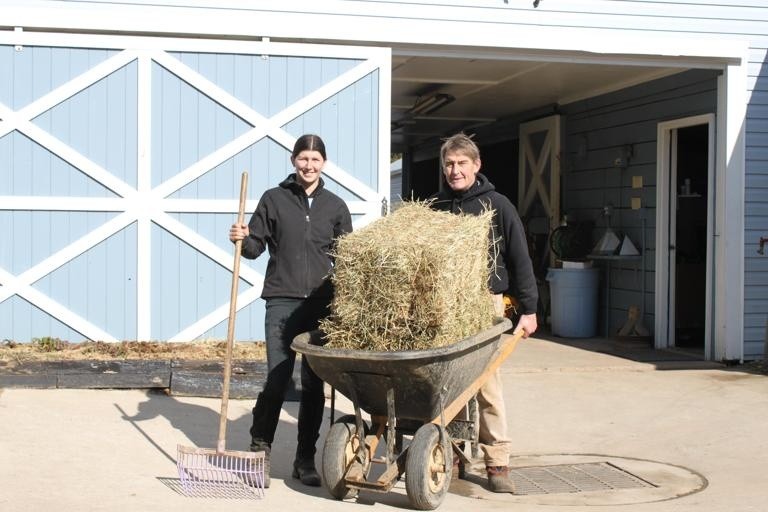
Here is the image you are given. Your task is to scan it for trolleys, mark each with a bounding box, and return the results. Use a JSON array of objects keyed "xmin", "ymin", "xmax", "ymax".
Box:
[{"xmin": 289, "ymin": 313, "xmax": 527, "ymax": 512}]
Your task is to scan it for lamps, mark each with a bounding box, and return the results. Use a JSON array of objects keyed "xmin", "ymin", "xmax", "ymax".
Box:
[{"xmin": 402, "ymin": 91, "xmax": 455, "ymax": 116}]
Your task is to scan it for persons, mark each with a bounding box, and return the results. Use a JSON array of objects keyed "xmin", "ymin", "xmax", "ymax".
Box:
[
  {"xmin": 428, "ymin": 132, "xmax": 539, "ymax": 491},
  {"xmin": 230, "ymin": 135, "xmax": 352, "ymax": 487}
]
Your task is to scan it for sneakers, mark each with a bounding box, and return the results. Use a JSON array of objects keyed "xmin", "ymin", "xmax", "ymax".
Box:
[
  {"xmin": 485, "ymin": 466, "xmax": 515, "ymax": 493},
  {"xmin": 293, "ymin": 467, "xmax": 322, "ymax": 485},
  {"xmin": 248, "ymin": 455, "xmax": 270, "ymax": 488}
]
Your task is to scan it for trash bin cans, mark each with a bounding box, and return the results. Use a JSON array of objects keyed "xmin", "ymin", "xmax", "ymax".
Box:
[{"xmin": 545, "ymin": 268, "xmax": 606, "ymax": 338}]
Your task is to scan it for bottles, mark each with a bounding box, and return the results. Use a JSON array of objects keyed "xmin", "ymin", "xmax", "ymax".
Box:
[{"xmin": 685, "ymin": 179, "xmax": 691, "ymax": 195}]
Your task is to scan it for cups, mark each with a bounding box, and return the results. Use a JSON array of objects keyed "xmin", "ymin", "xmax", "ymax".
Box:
[{"xmin": 681, "ymin": 186, "xmax": 685, "ymax": 195}]
